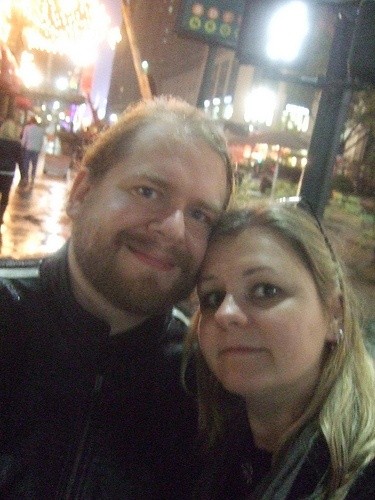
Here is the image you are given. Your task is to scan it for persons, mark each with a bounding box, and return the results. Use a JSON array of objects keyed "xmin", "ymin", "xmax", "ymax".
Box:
[
  {"xmin": 1, "ymin": 116, "xmax": 44, "ymax": 229},
  {"xmin": 180, "ymin": 203, "xmax": 375, "ymax": 500},
  {"xmin": 0, "ymin": 96, "xmax": 251, "ymax": 500}
]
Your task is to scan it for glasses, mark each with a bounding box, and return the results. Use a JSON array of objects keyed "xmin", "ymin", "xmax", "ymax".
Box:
[{"xmin": 276, "ymin": 196, "xmax": 345, "ymax": 300}]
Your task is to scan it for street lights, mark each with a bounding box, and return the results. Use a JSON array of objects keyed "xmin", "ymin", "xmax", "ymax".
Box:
[{"xmin": 257, "ymin": 1, "xmax": 366, "ymax": 223}]
